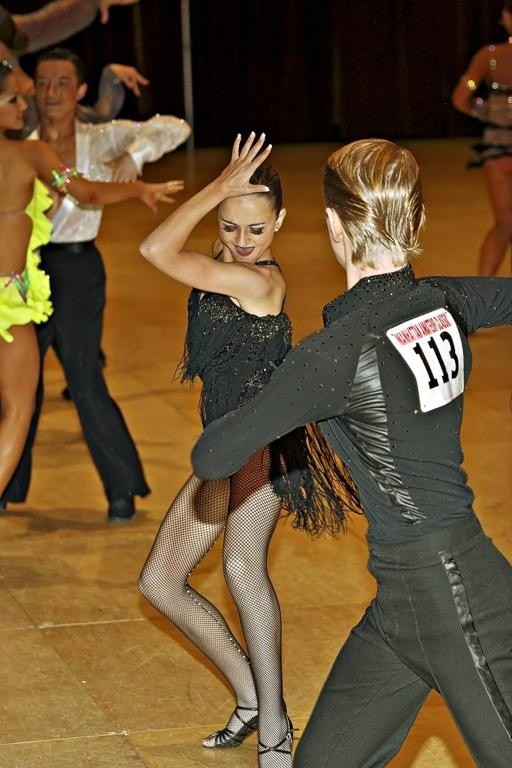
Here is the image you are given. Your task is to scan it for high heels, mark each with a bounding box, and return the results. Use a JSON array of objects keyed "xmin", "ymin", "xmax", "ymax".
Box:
[{"xmin": 107, "ymin": 481, "xmax": 150, "ymax": 521}]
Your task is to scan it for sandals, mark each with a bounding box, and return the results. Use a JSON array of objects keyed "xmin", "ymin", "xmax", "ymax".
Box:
[
  {"xmin": 205, "ymin": 702, "xmax": 262, "ymax": 750},
  {"xmin": 259, "ymin": 721, "xmax": 294, "ymax": 766}
]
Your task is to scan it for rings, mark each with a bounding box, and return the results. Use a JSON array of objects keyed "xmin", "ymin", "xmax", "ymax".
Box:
[
  {"xmin": 169, "ymin": 187, "xmax": 174, "ymax": 192},
  {"xmin": 167, "ymin": 181, "xmax": 174, "ymax": 186}
]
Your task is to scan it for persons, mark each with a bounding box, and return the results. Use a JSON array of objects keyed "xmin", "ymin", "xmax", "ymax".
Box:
[
  {"xmin": 134, "ymin": 129, "xmax": 303, "ymax": 768},
  {"xmin": 0, "ymin": 44, "xmax": 151, "ymax": 143},
  {"xmin": 451, "ymin": 7, "xmax": 510, "ymax": 279},
  {"xmin": 0, "ymin": 3, "xmax": 133, "ymax": 64},
  {"xmin": 193, "ymin": 139, "xmax": 510, "ymax": 767}
]
